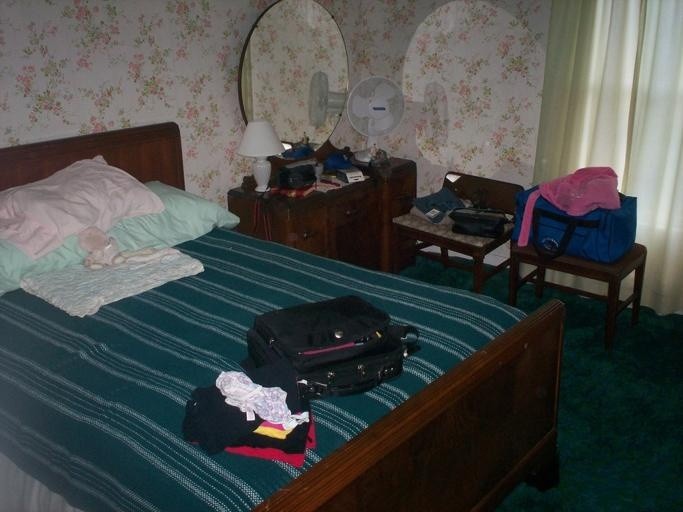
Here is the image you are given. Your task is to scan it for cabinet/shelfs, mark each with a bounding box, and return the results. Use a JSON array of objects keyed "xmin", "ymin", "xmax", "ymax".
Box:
[{"xmin": 225, "ymin": 156, "xmax": 417, "ymax": 275}]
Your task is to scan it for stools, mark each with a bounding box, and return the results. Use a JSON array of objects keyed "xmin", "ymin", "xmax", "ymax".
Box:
[{"xmin": 508, "ymin": 240, "xmax": 647, "ymax": 342}]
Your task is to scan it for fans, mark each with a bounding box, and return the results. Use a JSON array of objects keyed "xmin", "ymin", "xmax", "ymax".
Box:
[
  {"xmin": 344, "ymin": 75, "xmax": 405, "ymax": 167},
  {"xmin": 307, "ymin": 70, "xmax": 348, "ymax": 127}
]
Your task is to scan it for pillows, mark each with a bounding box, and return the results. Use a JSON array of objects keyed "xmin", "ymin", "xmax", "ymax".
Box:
[
  {"xmin": 0, "ymin": 180, "xmax": 240, "ymax": 300},
  {"xmin": 0, "ymin": 150, "xmax": 166, "ymax": 263}
]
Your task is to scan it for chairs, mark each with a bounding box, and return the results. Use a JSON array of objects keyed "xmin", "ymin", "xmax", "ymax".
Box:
[{"xmin": 388, "ymin": 169, "xmax": 525, "ymax": 294}]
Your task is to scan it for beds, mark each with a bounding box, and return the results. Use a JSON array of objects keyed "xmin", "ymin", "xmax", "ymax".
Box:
[{"xmin": 0, "ymin": 120, "xmax": 567, "ymax": 510}]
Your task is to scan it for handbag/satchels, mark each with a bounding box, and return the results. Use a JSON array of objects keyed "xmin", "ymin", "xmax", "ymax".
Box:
[
  {"xmin": 510, "ymin": 185, "xmax": 636, "ymax": 265},
  {"xmin": 237, "ymin": 293, "xmax": 423, "ymax": 402}
]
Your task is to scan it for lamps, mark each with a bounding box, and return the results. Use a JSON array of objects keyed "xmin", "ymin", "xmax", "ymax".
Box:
[{"xmin": 237, "ymin": 120, "xmax": 286, "ymax": 193}]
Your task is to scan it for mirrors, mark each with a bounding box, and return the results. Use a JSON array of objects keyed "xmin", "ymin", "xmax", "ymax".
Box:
[{"xmin": 235, "ymin": 1, "xmax": 351, "ymax": 161}]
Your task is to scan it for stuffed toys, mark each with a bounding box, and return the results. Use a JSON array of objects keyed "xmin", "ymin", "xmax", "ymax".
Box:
[{"xmin": 85, "ymin": 236, "xmax": 177, "ymax": 269}]
